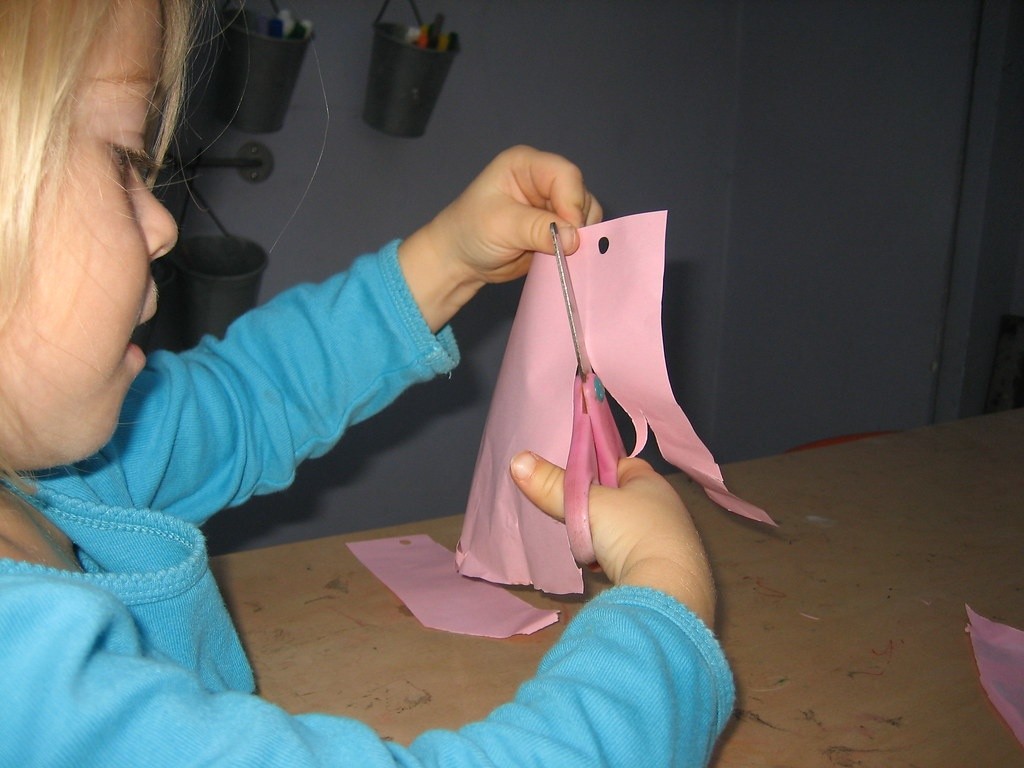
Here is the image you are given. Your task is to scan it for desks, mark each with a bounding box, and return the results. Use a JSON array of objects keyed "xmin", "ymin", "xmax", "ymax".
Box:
[{"xmin": 206, "ymin": 407, "xmax": 1024, "ymax": 768}]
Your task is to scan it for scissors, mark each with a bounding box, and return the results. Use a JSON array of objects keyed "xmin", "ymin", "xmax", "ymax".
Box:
[{"xmin": 548, "ymin": 223, "xmax": 629, "ymax": 572}]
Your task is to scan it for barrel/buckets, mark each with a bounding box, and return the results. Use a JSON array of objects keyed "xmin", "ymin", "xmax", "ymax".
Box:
[
  {"xmin": 218, "ymin": 10, "xmax": 314, "ymax": 133},
  {"xmin": 363, "ymin": 21, "xmax": 460, "ymax": 138},
  {"xmin": 175, "ymin": 235, "xmax": 268, "ymax": 354}
]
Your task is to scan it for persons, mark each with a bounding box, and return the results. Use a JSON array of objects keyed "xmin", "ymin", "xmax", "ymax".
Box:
[{"xmin": 0, "ymin": 0, "xmax": 741, "ymax": 768}]
